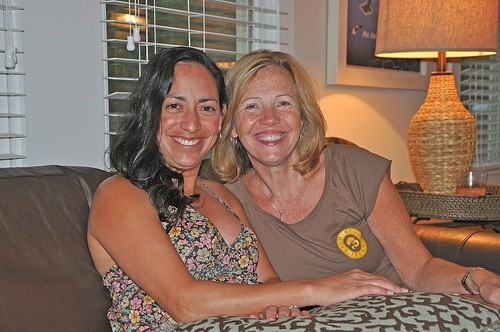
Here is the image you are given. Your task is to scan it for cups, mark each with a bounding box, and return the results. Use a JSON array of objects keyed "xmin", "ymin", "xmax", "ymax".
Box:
[{"xmin": 455, "ymin": 171, "xmax": 488, "ymax": 197}]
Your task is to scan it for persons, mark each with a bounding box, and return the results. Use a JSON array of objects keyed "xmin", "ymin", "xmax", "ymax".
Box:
[
  {"xmin": 212, "ymin": 50, "xmax": 500, "ymax": 312},
  {"xmin": 87, "ymin": 47, "xmax": 408, "ymax": 332}
]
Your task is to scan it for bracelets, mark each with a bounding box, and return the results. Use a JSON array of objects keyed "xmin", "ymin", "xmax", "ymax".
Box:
[{"xmin": 461, "ymin": 267, "xmax": 484, "ymax": 295}]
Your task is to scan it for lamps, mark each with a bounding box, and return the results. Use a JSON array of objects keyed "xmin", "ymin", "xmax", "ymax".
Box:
[{"xmin": 374, "ymin": 0, "xmax": 499, "ymax": 192}]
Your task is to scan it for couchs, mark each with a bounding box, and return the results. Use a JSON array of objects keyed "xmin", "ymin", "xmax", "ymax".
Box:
[{"xmin": 0, "ymin": 158, "xmax": 500, "ymax": 332}]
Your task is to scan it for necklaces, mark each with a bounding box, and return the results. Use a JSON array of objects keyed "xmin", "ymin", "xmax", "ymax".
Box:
[{"xmin": 253, "ymin": 170, "xmax": 303, "ymax": 222}]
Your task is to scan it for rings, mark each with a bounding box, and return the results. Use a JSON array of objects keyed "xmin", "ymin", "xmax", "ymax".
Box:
[{"xmin": 288, "ymin": 305, "xmax": 298, "ymax": 310}]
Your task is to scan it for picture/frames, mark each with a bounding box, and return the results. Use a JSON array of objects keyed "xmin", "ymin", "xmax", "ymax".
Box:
[{"xmin": 325, "ymin": 0, "xmax": 431, "ymax": 93}]
